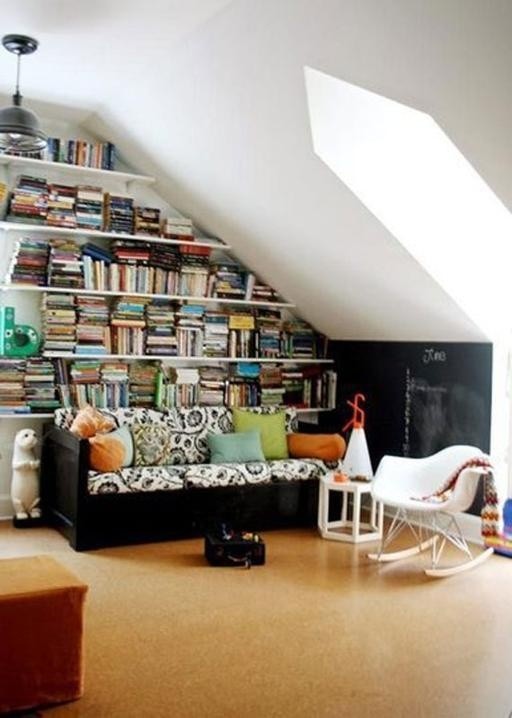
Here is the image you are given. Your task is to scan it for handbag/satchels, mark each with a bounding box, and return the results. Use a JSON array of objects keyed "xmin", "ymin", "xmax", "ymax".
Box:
[{"xmin": 206, "ymin": 533, "xmax": 264, "ymax": 566}]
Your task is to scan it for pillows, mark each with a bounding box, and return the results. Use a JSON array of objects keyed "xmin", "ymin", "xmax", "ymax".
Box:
[
  {"xmin": 67, "ymin": 403, "xmax": 112, "ymax": 438},
  {"xmin": 111, "ymin": 425, "xmax": 133, "ymax": 466},
  {"xmin": 88, "ymin": 432, "xmax": 126, "ymax": 474},
  {"xmin": 232, "ymin": 404, "xmax": 292, "ymax": 460},
  {"xmin": 287, "ymin": 432, "xmax": 347, "ymax": 462},
  {"xmin": 127, "ymin": 421, "xmax": 179, "ymax": 466},
  {"xmin": 203, "ymin": 429, "xmax": 265, "ymax": 466}
]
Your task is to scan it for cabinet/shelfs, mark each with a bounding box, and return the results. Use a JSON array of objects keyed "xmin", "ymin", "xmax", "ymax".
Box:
[{"xmin": 0, "ymin": 156, "xmax": 339, "ymax": 419}]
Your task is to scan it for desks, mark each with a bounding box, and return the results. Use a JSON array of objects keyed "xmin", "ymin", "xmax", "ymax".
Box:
[{"xmin": 314, "ymin": 469, "xmax": 382, "ymax": 545}]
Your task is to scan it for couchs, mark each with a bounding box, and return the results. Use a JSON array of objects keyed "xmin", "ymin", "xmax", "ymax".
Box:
[{"xmin": 40, "ymin": 407, "xmax": 350, "ymax": 553}]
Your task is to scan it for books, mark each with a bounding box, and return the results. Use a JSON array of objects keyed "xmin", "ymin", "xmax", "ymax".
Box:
[
  {"xmin": 1, "ymin": 138, "xmax": 116, "ymax": 171},
  {"xmin": 39, "ymin": 291, "xmax": 329, "ymax": 360},
  {"xmin": 0, "ymin": 356, "xmax": 336, "ymax": 415},
  {"xmin": 2, "ymin": 174, "xmax": 195, "ymax": 242},
  {"xmin": 6, "ymin": 238, "xmax": 281, "ymax": 303}
]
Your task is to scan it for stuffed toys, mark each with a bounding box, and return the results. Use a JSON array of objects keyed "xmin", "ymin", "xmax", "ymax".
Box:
[{"xmin": 10, "ymin": 428, "xmax": 41, "ymax": 519}]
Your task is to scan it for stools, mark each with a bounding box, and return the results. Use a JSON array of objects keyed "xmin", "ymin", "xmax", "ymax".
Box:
[{"xmin": 0, "ymin": 555, "xmax": 89, "ymax": 709}]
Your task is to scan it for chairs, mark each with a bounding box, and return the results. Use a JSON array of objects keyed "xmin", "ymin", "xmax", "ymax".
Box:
[{"xmin": 364, "ymin": 445, "xmax": 494, "ymax": 577}]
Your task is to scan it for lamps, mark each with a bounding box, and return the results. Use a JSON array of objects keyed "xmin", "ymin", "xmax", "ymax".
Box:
[{"xmin": 0, "ymin": 33, "xmax": 48, "ymax": 152}]
